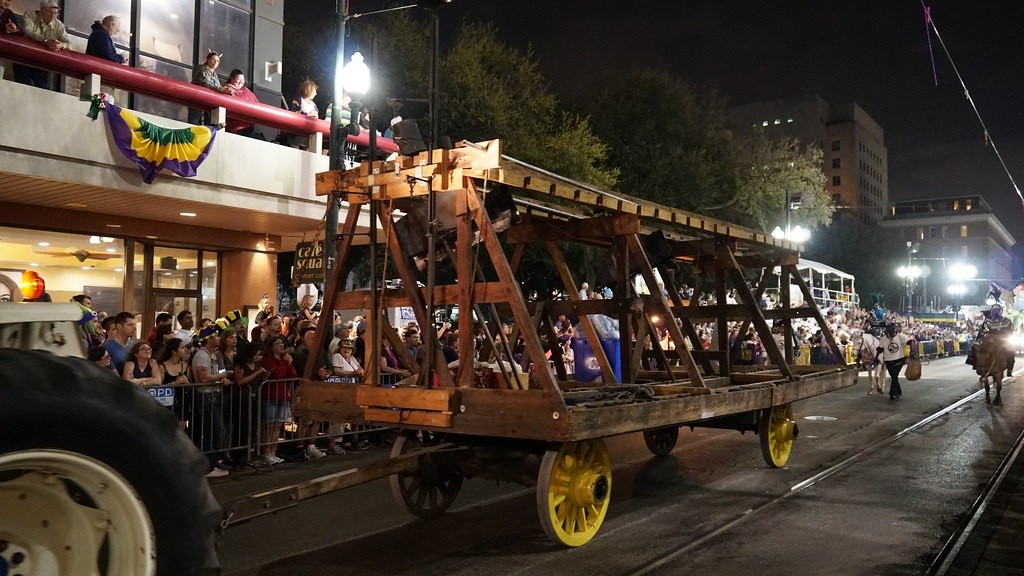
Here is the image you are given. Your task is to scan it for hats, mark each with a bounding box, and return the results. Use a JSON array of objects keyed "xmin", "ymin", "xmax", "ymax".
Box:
[
  {"xmin": 334, "ymin": 324, "xmax": 352, "ymax": 332},
  {"xmin": 338, "ymin": 339, "xmax": 354, "ymax": 347},
  {"xmin": 196, "ymin": 325, "xmax": 217, "ymax": 341},
  {"xmin": 226, "ymin": 310, "xmax": 242, "ymax": 325},
  {"xmin": 214, "ymin": 317, "xmax": 231, "ymax": 336}
]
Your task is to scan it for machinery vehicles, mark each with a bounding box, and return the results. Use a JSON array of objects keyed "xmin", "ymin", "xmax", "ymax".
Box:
[{"xmin": 0, "ymin": 269, "xmax": 234, "ymax": 576}]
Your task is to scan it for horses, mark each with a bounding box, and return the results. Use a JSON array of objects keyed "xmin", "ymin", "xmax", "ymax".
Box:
[{"xmin": 972, "ymin": 332, "xmax": 1015, "ymax": 406}]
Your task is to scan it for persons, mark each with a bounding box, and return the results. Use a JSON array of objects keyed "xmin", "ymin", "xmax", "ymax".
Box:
[
  {"xmin": 325, "ymin": 94, "xmax": 402, "ymax": 161},
  {"xmin": 84, "ymin": 15, "xmax": 127, "ymax": 104},
  {"xmin": 0, "ymin": 0, "xmax": 21, "ymax": 33},
  {"xmin": 73, "ymin": 282, "xmax": 658, "ymax": 472},
  {"xmin": 12, "ymin": 0, "xmax": 71, "ymax": 90},
  {"xmin": 188, "ymin": 51, "xmax": 232, "ymax": 125},
  {"xmin": 659, "ymin": 282, "xmax": 982, "ymax": 366},
  {"xmin": 877, "ymin": 322, "xmax": 916, "ymax": 399},
  {"xmin": 289, "ymin": 80, "xmax": 319, "ymax": 152},
  {"xmin": 970, "ymin": 304, "xmax": 1016, "ymax": 377},
  {"xmin": 223, "ymin": 69, "xmax": 261, "ymax": 137}
]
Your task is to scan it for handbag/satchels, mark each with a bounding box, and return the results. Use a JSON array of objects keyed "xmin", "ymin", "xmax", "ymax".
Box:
[{"xmin": 904, "ymin": 359, "xmax": 921, "ymax": 380}]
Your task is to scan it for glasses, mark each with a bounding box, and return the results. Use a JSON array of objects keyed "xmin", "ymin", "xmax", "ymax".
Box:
[
  {"xmin": 269, "ymin": 322, "xmax": 283, "ymax": 326},
  {"xmin": 101, "ymin": 355, "xmax": 110, "ymax": 360},
  {"xmin": 139, "ymin": 348, "xmax": 152, "ymax": 351}
]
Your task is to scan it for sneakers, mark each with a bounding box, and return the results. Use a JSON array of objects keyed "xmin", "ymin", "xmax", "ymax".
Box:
[
  {"xmin": 307, "ymin": 447, "xmax": 326, "ymax": 458},
  {"xmin": 272, "ymin": 452, "xmax": 284, "ymax": 464},
  {"xmin": 261, "ymin": 452, "xmax": 277, "ymax": 465}
]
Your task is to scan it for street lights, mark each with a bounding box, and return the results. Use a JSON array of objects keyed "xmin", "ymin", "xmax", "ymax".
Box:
[
  {"xmin": 899, "ymin": 263, "xmax": 922, "ymax": 317},
  {"xmin": 323, "ymin": 50, "xmax": 372, "ymax": 349}
]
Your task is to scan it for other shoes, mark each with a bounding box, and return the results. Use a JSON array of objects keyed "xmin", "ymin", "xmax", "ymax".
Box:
[
  {"xmin": 1007, "ymin": 369, "xmax": 1012, "ymax": 377},
  {"xmin": 889, "ymin": 392, "xmax": 896, "ymax": 400},
  {"xmin": 208, "ymin": 467, "xmax": 229, "ymax": 477},
  {"xmin": 326, "ymin": 445, "xmax": 344, "ymax": 455},
  {"xmin": 895, "ymin": 393, "xmax": 901, "ymax": 398},
  {"xmin": 350, "ymin": 441, "xmax": 369, "ymax": 450}
]
